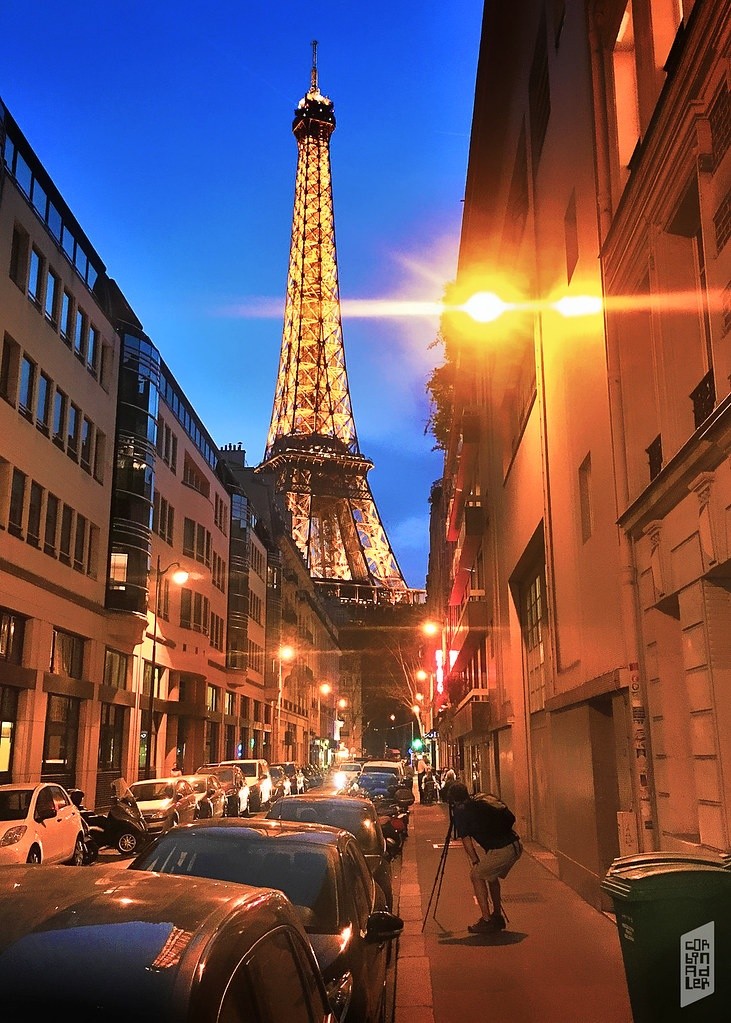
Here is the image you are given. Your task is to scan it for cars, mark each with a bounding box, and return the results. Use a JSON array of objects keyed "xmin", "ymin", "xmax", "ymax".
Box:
[
  {"xmin": 0, "ymin": 788, "xmax": 86, "ymax": 867},
  {"xmin": 124, "ymin": 818, "xmax": 405, "ymax": 1023},
  {"xmin": 0, "ymin": 864, "xmax": 340, "ymax": 1023},
  {"xmin": 156, "ymin": 773, "xmax": 229, "ymax": 818},
  {"xmin": 124, "ymin": 779, "xmax": 200, "ymax": 855},
  {"xmin": 268, "ymin": 761, "xmax": 415, "ymax": 857},
  {"xmin": 264, "ymin": 792, "xmax": 398, "ymax": 877}
]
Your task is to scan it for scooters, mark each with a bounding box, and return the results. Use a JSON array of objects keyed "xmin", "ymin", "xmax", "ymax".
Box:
[{"xmin": 64, "ymin": 776, "xmax": 148, "ymax": 864}]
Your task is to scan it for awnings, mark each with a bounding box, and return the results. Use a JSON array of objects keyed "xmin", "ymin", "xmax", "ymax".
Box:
[{"xmin": 388, "ymin": 749, "xmax": 400, "ymax": 754}]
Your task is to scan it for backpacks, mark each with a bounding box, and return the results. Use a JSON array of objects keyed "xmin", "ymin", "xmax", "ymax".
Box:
[{"xmin": 468, "ymin": 792, "xmax": 516, "ymax": 839}]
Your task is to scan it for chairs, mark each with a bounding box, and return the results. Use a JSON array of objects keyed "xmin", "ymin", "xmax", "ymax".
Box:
[{"xmin": 299, "ymin": 808, "xmax": 318, "ymax": 822}]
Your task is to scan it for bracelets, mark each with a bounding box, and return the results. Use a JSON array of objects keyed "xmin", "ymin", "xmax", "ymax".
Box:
[{"xmin": 473, "ymin": 860, "xmax": 480, "ymax": 865}]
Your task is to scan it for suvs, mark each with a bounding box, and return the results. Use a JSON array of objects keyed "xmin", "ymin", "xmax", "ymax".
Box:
[
  {"xmin": 194, "ymin": 764, "xmax": 251, "ymax": 819},
  {"xmin": 218, "ymin": 758, "xmax": 273, "ymax": 811}
]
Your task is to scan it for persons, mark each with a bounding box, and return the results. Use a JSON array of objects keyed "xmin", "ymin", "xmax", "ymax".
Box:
[
  {"xmin": 411, "ymin": 753, "xmax": 457, "ymax": 805},
  {"xmin": 447, "ymin": 781, "xmax": 523, "ymax": 935}
]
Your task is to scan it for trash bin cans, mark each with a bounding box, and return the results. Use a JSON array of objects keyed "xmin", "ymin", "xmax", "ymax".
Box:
[{"xmin": 602, "ymin": 852, "xmax": 731, "ymax": 1023}]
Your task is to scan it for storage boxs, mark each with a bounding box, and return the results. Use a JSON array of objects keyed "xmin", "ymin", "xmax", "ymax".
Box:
[
  {"xmin": 66, "ymin": 788, "xmax": 84, "ymax": 806},
  {"xmin": 394, "ymin": 789, "xmax": 414, "ymax": 806},
  {"xmin": 388, "ymin": 784, "xmax": 401, "ymax": 794}
]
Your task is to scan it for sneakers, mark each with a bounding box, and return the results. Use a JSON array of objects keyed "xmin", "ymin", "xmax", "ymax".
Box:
[
  {"xmin": 468, "ymin": 917, "xmax": 494, "ymax": 933},
  {"xmin": 490, "ymin": 911, "xmax": 507, "ymax": 928}
]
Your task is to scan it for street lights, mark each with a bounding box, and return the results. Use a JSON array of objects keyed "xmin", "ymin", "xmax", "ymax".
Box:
[{"xmin": 143, "ymin": 553, "xmax": 191, "ymax": 782}]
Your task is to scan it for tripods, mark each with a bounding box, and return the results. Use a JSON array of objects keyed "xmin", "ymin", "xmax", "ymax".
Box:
[{"xmin": 422, "ymin": 804, "xmax": 509, "ymax": 933}]
